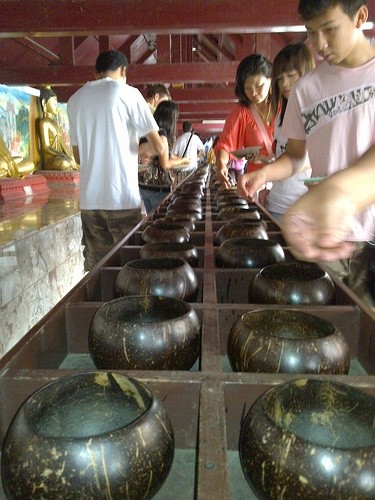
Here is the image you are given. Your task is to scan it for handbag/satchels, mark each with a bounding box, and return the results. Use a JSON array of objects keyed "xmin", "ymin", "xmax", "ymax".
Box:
[{"xmin": 138, "ymin": 128, "xmax": 176, "ymax": 189}]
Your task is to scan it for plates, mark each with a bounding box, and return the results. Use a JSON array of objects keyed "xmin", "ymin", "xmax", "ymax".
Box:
[
  {"xmin": 229, "ymin": 146, "xmax": 263, "ymax": 157},
  {"xmin": 298, "ymin": 177, "xmax": 328, "ymax": 187}
]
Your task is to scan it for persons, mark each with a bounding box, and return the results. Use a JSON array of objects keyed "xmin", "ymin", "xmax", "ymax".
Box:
[
  {"xmin": 38, "ymin": 86, "xmax": 80, "ymax": 170},
  {"xmin": 0, "ymin": 134, "xmax": 35, "ymax": 178},
  {"xmin": 66, "ymin": 0, "xmax": 375, "ymax": 313}
]
